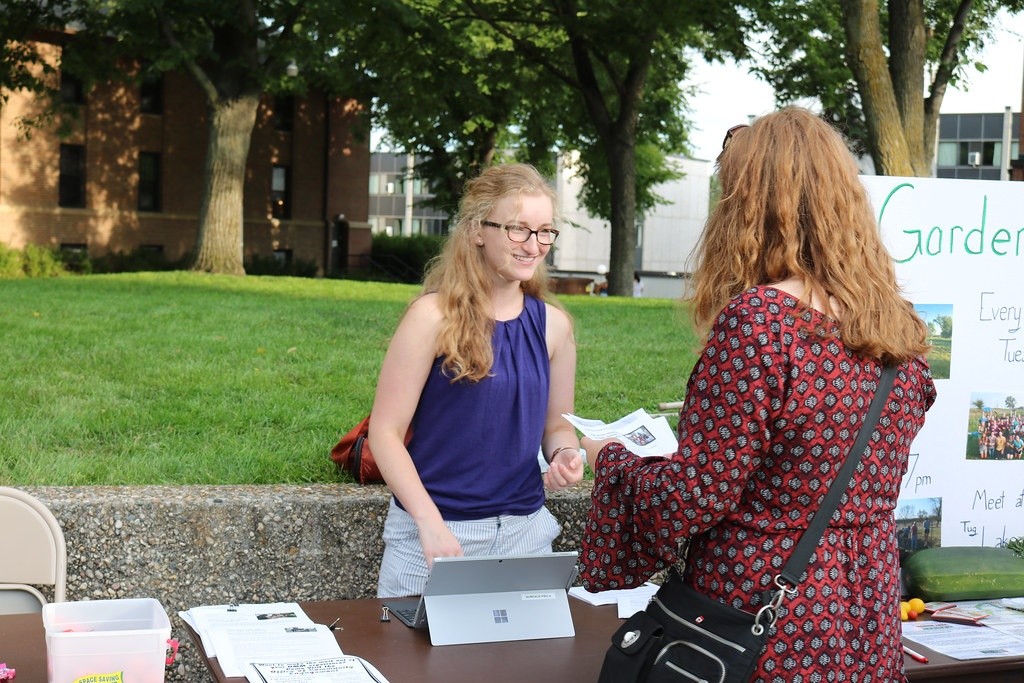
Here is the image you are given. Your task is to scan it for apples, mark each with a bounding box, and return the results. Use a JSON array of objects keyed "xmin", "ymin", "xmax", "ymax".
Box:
[{"xmin": 901, "ymin": 598, "xmax": 925, "ymax": 621}]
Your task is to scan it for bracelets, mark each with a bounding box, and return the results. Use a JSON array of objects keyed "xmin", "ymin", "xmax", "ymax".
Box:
[{"xmin": 550, "ymin": 446, "xmax": 580, "ymax": 464}]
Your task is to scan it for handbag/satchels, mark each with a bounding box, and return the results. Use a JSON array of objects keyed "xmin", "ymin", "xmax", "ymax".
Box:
[
  {"xmin": 596, "ymin": 564, "xmax": 772, "ymax": 683},
  {"xmin": 330, "ymin": 408, "xmax": 414, "ymax": 486}
]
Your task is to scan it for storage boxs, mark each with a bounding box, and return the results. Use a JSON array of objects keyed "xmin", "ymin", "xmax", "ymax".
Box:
[{"xmin": 41, "ymin": 597, "xmax": 179, "ymax": 683}]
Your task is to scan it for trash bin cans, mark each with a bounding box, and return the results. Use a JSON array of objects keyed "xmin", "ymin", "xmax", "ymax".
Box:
[
  {"xmin": 272, "ymin": 249, "xmax": 293, "ymax": 275},
  {"xmin": 138, "ymin": 242, "xmax": 166, "ymax": 275},
  {"xmin": 62, "ymin": 242, "xmax": 90, "ymax": 277}
]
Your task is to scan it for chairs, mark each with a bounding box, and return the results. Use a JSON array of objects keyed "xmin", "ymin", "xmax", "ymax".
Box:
[{"xmin": 0, "ymin": 485, "xmax": 67, "ymax": 615}]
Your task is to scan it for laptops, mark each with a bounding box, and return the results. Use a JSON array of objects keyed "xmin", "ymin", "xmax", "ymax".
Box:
[{"xmin": 384, "ymin": 551, "xmax": 578, "ymax": 628}]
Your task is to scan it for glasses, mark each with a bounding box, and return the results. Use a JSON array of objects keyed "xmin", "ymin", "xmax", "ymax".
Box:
[
  {"xmin": 722, "ymin": 124, "xmax": 748, "ymax": 149},
  {"xmin": 479, "ymin": 218, "xmax": 560, "ymax": 247}
]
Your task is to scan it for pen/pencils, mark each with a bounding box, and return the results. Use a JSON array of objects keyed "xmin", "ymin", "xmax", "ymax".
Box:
[
  {"xmin": 658, "ymin": 401, "xmax": 685, "ymax": 412},
  {"xmin": 903, "ymin": 645, "xmax": 929, "ymax": 665}
]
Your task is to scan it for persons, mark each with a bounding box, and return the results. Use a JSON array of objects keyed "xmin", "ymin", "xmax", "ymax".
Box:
[
  {"xmin": 896, "ymin": 515, "xmax": 931, "ymax": 549},
  {"xmin": 581, "ymin": 104, "xmax": 939, "ymax": 683},
  {"xmin": 364, "ymin": 159, "xmax": 587, "ymax": 597},
  {"xmin": 977, "ymin": 411, "xmax": 1024, "ymax": 460}
]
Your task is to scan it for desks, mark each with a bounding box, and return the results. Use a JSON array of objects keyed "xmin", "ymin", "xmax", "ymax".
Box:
[
  {"xmin": 0, "ymin": 612, "xmax": 48, "ymax": 683},
  {"xmin": 181, "ymin": 595, "xmax": 1024, "ymax": 683}
]
